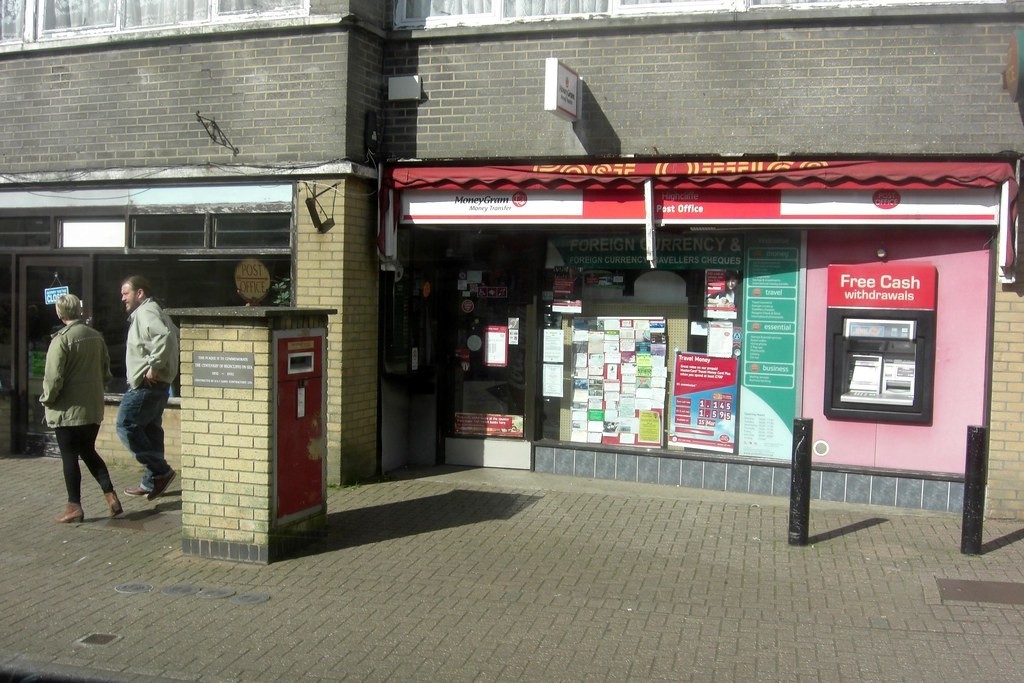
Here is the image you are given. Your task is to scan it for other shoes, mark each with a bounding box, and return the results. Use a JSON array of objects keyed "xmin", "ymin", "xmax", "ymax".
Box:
[
  {"xmin": 148, "ymin": 471, "xmax": 176, "ymax": 501},
  {"xmin": 124, "ymin": 485, "xmax": 151, "ymax": 496}
]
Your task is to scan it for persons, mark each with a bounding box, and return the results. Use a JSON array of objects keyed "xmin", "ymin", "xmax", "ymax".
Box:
[
  {"xmin": 114, "ymin": 273, "xmax": 180, "ymax": 502},
  {"xmin": 38, "ymin": 294, "xmax": 124, "ymax": 523},
  {"xmin": 706, "ymin": 270, "xmax": 739, "ymax": 308}
]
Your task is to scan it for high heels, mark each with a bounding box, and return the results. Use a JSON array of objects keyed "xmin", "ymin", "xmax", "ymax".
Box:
[
  {"xmin": 55, "ymin": 502, "xmax": 84, "ymax": 524},
  {"xmin": 104, "ymin": 492, "xmax": 122, "ymax": 518}
]
